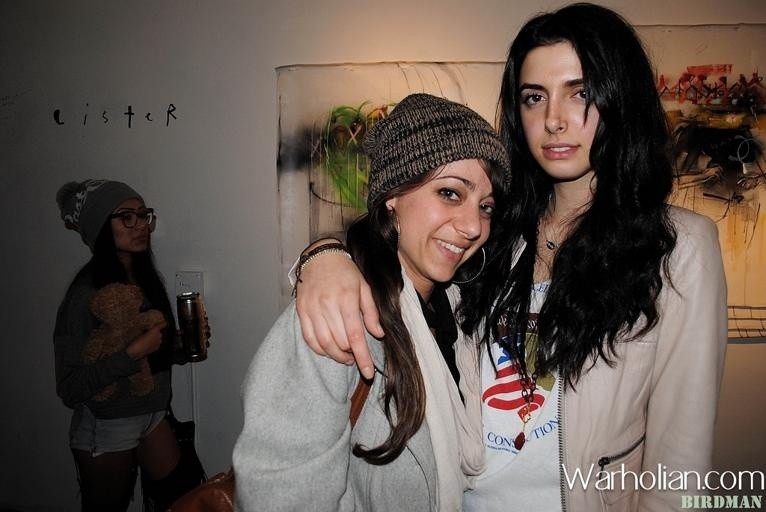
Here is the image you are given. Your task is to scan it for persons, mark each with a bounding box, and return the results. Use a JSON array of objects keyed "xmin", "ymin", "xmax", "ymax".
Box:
[
  {"xmin": 53, "ymin": 180, "xmax": 211, "ymax": 512},
  {"xmin": 233, "ymin": 0, "xmax": 727, "ymax": 512}
]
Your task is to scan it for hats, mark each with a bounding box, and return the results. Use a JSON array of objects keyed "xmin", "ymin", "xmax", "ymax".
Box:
[
  {"xmin": 56, "ymin": 178, "xmax": 144, "ymax": 247},
  {"xmin": 361, "ymin": 92, "xmax": 513, "ymax": 213}
]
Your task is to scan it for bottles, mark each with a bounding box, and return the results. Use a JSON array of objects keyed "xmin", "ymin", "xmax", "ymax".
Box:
[{"xmin": 177, "ymin": 291, "xmax": 207, "ymax": 363}]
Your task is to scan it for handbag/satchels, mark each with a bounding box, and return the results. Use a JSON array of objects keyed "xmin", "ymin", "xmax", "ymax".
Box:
[{"xmin": 166, "ymin": 471, "xmax": 236, "ymax": 512}]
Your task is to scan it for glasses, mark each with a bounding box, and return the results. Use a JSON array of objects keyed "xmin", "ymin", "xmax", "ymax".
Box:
[{"xmin": 107, "ymin": 205, "xmax": 155, "ymax": 230}]
